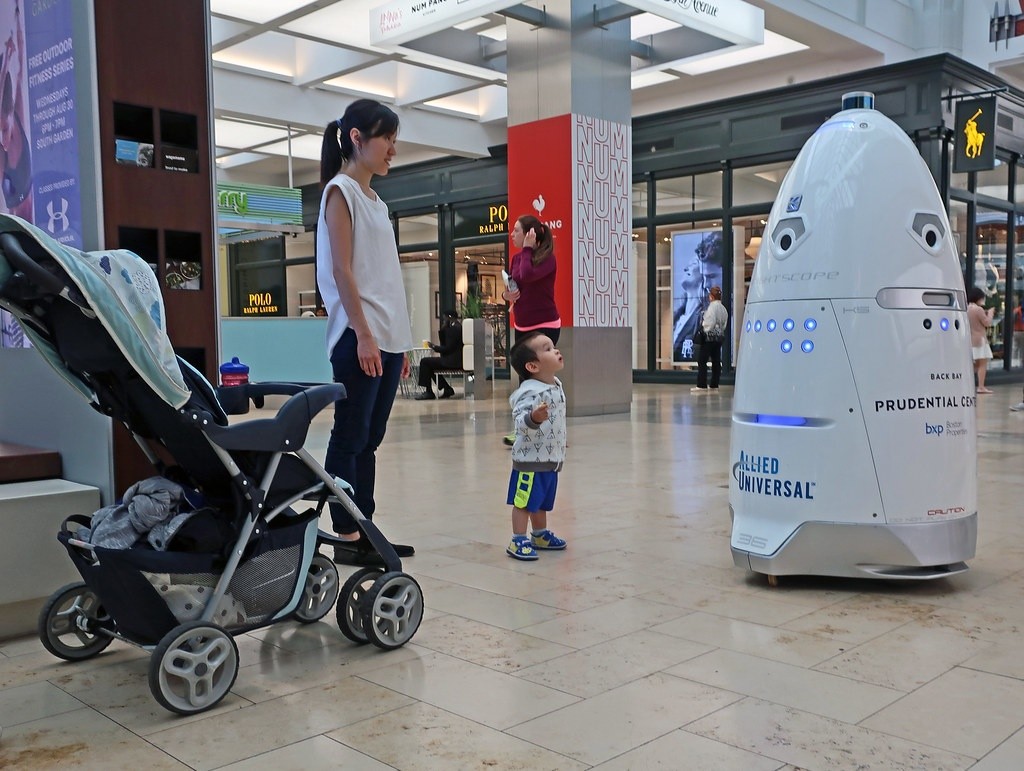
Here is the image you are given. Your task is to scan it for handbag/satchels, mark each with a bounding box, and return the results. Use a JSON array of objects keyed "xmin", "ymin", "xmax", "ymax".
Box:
[{"xmin": 693, "ymin": 312, "xmax": 707, "ymax": 344}]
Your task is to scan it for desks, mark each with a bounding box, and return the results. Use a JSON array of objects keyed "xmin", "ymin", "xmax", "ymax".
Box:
[{"xmin": 399, "ymin": 348, "xmax": 435, "ymax": 399}]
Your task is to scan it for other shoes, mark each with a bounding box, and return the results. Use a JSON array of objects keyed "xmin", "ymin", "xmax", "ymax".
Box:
[
  {"xmin": 690, "ymin": 387, "xmax": 708, "ymax": 391},
  {"xmin": 977, "ymin": 387, "xmax": 992, "ymax": 393},
  {"xmin": 334, "ymin": 540, "xmax": 386, "ymax": 566},
  {"xmin": 415, "ymin": 392, "xmax": 435, "ymax": 400},
  {"xmin": 708, "ymin": 386, "xmax": 719, "ymax": 390},
  {"xmin": 504, "ymin": 430, "xmax": 516, "ymax": 445},
  {"xmin": 1010, "ymin": 402, "xmax": 1024, "ymax": 411},
  {"xmin": 438, "ymin": 387, "xmax": 454, "ymax": 398},
  {"xmin": 389, "ymin": 542, "xmax": 415, "ymax": 556}
]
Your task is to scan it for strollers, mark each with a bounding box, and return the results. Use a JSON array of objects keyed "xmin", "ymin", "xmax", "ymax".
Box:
[{"xmin": 0, "ymin": 214, "xmax": 424, "ymax": 715}]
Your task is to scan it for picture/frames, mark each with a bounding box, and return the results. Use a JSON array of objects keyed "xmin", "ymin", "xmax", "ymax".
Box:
[
  {"xmin": 435, "ymin": 291, "xmax": 463, "ymax": 319},
  {"xmin": 480, "ymin": 273, "xmax": 496, "ymax": 300}
]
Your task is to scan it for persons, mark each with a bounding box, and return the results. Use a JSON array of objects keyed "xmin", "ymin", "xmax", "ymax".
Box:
[
  {"xmin": 506, "ymin": 331, "xmax": 567, "ymax": 561},
  {"xmin": 415, "ymin": 310, "xmax": 463, "ymax": 400},
  {"xmin": 690, "ymin": 287, "xmax": 728, "ymax": 391},
  {"xmin": 503, "ymin": 215, "xmax": 561, "ymax": 445},
  {"xmin": 966, "ymin": 287, "xmax": 995, "ymax": 392},
  {"xmin": 317, "ymin": 100, "xmax": 415, "ymax": 567},
  {"xmin": 1013, "ymin": 294, "xmax": 1024, "ymax": 359},
  {"xmin": 0, "ymin": 6, "xmax": 33, "ymax": 222}
]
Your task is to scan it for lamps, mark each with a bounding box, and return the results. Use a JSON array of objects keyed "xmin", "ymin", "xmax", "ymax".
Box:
[{"xmin": 744, "ymin": 220, "xmax": 762, "ymax": 260}]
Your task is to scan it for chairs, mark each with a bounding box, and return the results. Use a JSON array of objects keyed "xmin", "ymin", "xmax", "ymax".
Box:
[{"xmin": 433, "ymin": 318, "xmax": 488, "ymax": 401}]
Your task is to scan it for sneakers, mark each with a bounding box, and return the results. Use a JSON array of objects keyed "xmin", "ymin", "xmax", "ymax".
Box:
[
  {"xmin": 530, "ymin": 530, "xmax": 567, "ymax": 549},
  {"xmin": 506, "ymin": 538, "xmax": 540, "ymax": 560}
]
